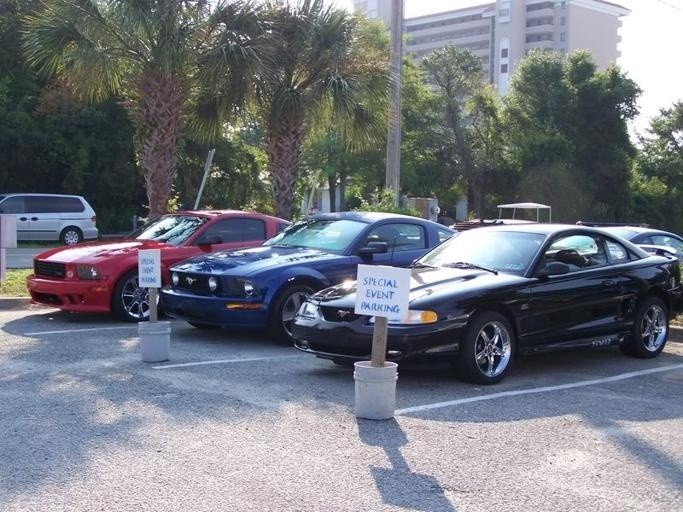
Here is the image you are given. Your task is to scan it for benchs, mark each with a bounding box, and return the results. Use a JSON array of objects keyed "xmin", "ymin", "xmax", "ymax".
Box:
[{"xmin": 546, "ymin": 253, "xmax": 603, "ymax": 268}]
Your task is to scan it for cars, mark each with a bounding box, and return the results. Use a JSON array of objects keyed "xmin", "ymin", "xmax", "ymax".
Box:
[
  {"xmin": 550, "ymin": 226, "xmax": 683, "ymax": 292},
  {"xmin": 289, "ymin": 223, "xmax": 683, "ymax": 384},
  {"xmin": 166, "ymin": 213, "xmax": 463, "ymax": 345},
  {"xmin": 27, "ymin": 209, "xmax": 292, "ymax": 325}
]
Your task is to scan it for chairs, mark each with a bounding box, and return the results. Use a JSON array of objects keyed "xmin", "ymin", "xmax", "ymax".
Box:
[{"xmin": 542, "ymin": 262, "xmax": 576, "ymax": 276}]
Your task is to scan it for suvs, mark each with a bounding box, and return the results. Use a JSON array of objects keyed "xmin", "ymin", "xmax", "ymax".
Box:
[{"xmin": 0, "ymin": 192, "xmax": 99, "ymax": 246}]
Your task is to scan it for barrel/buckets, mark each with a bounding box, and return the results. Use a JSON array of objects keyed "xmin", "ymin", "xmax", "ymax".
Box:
[
  {"xmin": 137, "ymin": 321, "xmax": 172, "ymax": 363},
  {"xmin": 352, "ymin": 361, "xmax": 399, "ymax": 421}
]
